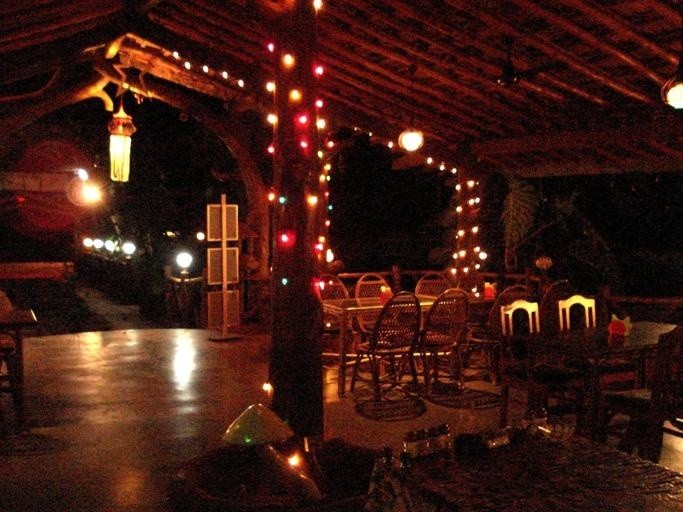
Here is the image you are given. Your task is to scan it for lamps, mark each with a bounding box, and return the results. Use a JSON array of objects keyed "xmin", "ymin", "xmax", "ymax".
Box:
[
  {"xmin": 661, "ymin": 78, "xmax": 683, "ymax": 109},
  {"xmin": 121, "ymin": 240, "xmax": 137, "ymax": 261},
  {"xmin": 78, "ymin": 163, "xmax": 101, "ymax": 181},
  {"xmin": 104, "ymin": 88, "xmax": 134, "ymax": 184},
  {"xmin": 175, "ymin": 251, "xmax": 193, "ymax": 280}
]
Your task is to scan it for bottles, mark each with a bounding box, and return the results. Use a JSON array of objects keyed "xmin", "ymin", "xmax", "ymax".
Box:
[{"xmin": 402, "ymin": 423, "xmax": 450, "ymax": 465}]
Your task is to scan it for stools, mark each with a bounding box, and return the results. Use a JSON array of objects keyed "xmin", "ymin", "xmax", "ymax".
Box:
[{"xmin": 0, "ymin": 292, "xmax": 38, "ymax": 433}]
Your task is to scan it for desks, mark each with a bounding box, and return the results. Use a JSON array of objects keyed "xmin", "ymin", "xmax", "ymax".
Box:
[{"xmin": 168, "ymin": 424, "xmax": 683, "ymax": 510}]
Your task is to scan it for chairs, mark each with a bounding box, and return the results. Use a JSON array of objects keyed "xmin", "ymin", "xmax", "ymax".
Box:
[
  {"xmin": 320, "ymin": 260, "xmax": 493, "ymax": 410},
  {"xmin": 485, "ymin": 277, "xmax": 683, "ymax": 443}
]
